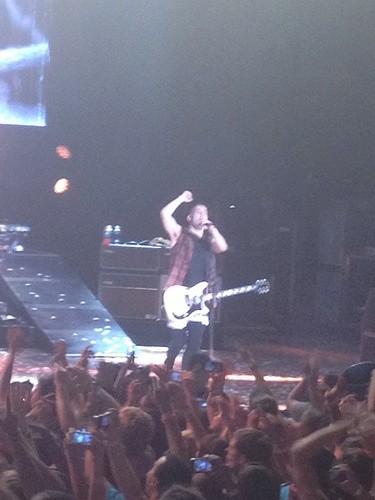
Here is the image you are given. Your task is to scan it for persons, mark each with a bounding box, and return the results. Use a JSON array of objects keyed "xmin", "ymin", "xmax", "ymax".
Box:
[
  {"xmin": 158, "ymin": 190, "xmax": 228, "ymax": 380},
  {"xmin": 0, "ymin": 326, "xmax": 375, "ymax": 500}
]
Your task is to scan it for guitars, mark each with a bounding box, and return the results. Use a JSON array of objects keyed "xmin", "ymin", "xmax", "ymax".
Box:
[{"xmin": 162, "ymin": 277, "xmax": 272, "ymax": 330}]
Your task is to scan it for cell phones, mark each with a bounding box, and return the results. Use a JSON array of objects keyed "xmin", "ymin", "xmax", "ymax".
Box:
[
  {"xmin": 189, "ymin": 458, "xmax": 210, "ymax": 473},
  {"xmin": 205, "ymin": 361, "xmax": 222, "ymax": 372},
  {"xmin": 70, "ymin": 432, "xmax": 93, "ymax": 446},
  {"xmin": 93, "ymin": 411, "xmax": 111, "ymax": 427},
  {"xmin": 278, "ymin": 482, "xmax": 291, "ymax": 500},
  {"xmin": 170, "ymin": 370, "xmax": 185, "ymax": 382}
]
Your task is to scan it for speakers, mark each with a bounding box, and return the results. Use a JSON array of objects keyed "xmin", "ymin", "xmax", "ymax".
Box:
[{"xmin": 96, "ymin": 245, "xmax": 164, "ymax": 322}]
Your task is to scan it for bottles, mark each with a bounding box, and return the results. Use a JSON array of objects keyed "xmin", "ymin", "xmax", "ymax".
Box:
[
  {"xmin": 103, "ymin": 224, "xmax": 113, "ymax": 244},
  {"xmin": 113, "ymin": 224, "xmax": 121, "ymax": 244}
]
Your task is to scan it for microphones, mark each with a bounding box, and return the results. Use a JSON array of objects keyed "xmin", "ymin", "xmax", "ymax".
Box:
[{"xmin": 204, "ymin": 223, "xmax": 214, "ymax": 226}]
